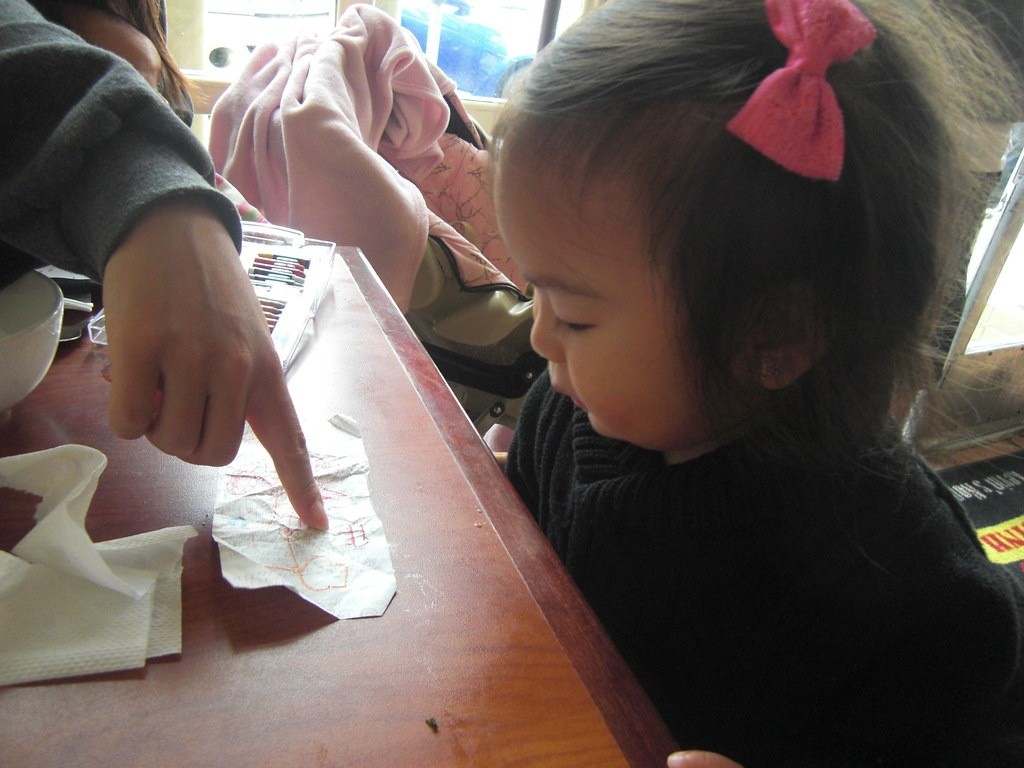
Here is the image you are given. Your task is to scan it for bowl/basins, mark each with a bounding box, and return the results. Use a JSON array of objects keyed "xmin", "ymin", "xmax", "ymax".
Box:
[{"xmin": 0, "ymin": 269, "xmax": 65, "ymax": 414}]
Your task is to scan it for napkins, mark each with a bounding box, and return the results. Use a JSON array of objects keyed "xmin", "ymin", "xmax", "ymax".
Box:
[{"xmin": 0, "ymin": 443, "xmax": 199, "ymax": 687}]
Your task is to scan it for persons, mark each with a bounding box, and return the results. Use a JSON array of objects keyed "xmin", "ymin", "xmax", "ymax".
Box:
[
  {"xmin": 491, "ymin": 0, "xmax": 1024, "ymax": 767},
  {"xmin": 28, "ymin": 0, "xmax": 212, "ymax": 126},
  {"xmin": 1, "ymin": 0, "xmax": 333, "ymax": 535}
]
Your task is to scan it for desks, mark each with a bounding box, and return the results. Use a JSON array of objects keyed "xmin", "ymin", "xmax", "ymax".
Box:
[{"xmin": 0, "ymin": 246, "xmax": 682, "ymax": 768}]
